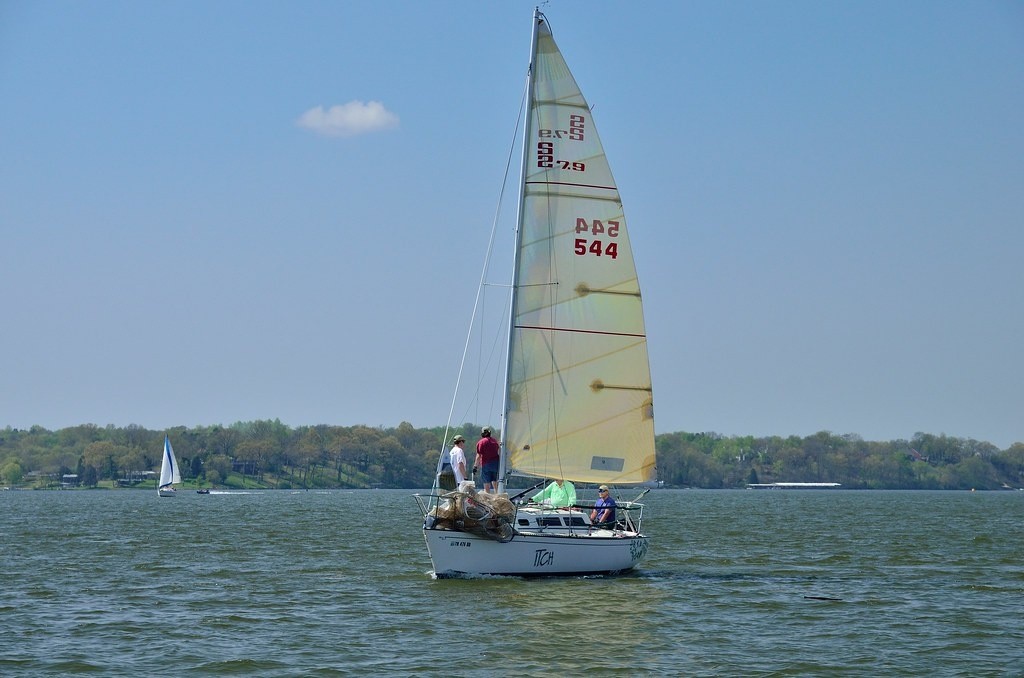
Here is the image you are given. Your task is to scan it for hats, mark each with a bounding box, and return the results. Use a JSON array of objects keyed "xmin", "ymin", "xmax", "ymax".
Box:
[
  {"xmin": 599, "ymin": 485, "xmax": 608, "ymax": 490},
  {"xmin": 454, "ymin": 435, "xmax": 466, "ymax": 442},
  {"xmin": 482, "ymin": 427, "xmax": 491, "ymax": 433}
]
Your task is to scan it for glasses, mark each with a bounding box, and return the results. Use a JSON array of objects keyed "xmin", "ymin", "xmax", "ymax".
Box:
[
  {"xmin": 462, "ymin": 440, "xmax": 464, "ymax": 443},
  {"xmin": 599, "ymin": 489, "xmax": 607, "ymax": 493}
]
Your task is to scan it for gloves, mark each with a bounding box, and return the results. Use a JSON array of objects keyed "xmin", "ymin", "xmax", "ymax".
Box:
[{"xmin": 473, "ymin": 466, "xmax": 477, "ymax": 475}]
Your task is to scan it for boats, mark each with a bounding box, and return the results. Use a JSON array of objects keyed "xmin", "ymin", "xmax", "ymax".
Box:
[
  {"xmin": 3, "ymin": 487, "xmax": 10, "ymax": 492},
  {"xmin": 197, "ymin": 489, "xmax": 210, "ymax": 495}
]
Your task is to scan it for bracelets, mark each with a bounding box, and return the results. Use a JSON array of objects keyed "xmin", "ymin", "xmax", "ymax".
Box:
[{"xmin": 599, "ymin": 521, "xmax": 603, "ymax": 523}]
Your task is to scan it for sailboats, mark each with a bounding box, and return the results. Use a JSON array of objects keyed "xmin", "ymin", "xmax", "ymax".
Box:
[
  {"xmin": 413, "ymin": 5, "xmax": 657, "ymax": 581},
  {"xmin": 158, "ymin": 434, "xmax": 181, "ymax": 497}
]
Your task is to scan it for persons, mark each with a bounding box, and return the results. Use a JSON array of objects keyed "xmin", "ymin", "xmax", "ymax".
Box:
[
  {"xmin": 449, "ymin": 435, "xmax": 468, "ymax": 486},
  {"xmin": 472, "ymin": 426, "xmax": 499, "ymax": 494},
  {"xmin": 590, "ymin": 485, "xmax": 616, "ymax": 531},
  {"xmin": 532, "ymin": 477, "xmax": 576, "ymax": 508}
]
